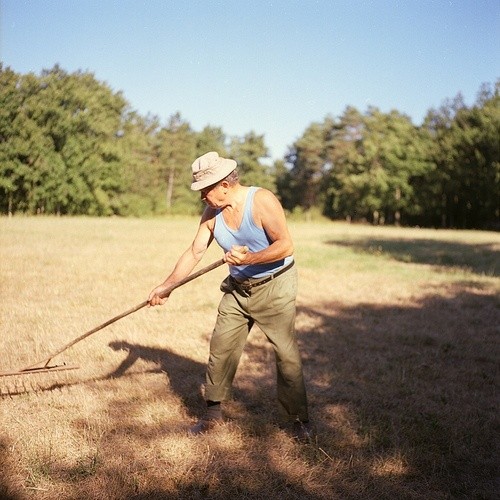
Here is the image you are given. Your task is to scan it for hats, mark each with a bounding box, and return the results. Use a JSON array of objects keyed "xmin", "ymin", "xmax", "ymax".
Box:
[{"xmin": 189, "ymin": 152, "xmax": 237, "ymax": 191}]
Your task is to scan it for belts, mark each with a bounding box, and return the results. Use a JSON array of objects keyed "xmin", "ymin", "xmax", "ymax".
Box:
[{"xmin": 231, "ymin": 260, "xmax": 295, "ymax": 290}]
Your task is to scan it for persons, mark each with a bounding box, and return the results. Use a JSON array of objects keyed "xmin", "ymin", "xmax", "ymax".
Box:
[{"xmin": 149, "ymin": 150, "xmax": 310, "ymax": 435}]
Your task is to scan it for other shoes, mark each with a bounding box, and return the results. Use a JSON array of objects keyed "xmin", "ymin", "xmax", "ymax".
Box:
[
  {"xmin": 189, "ymin": 418, "xmax": 222, "ymax": 434},
  {"xmin": 292, "ymin": 423, "xmax": 310, "ymax": 445}
]
